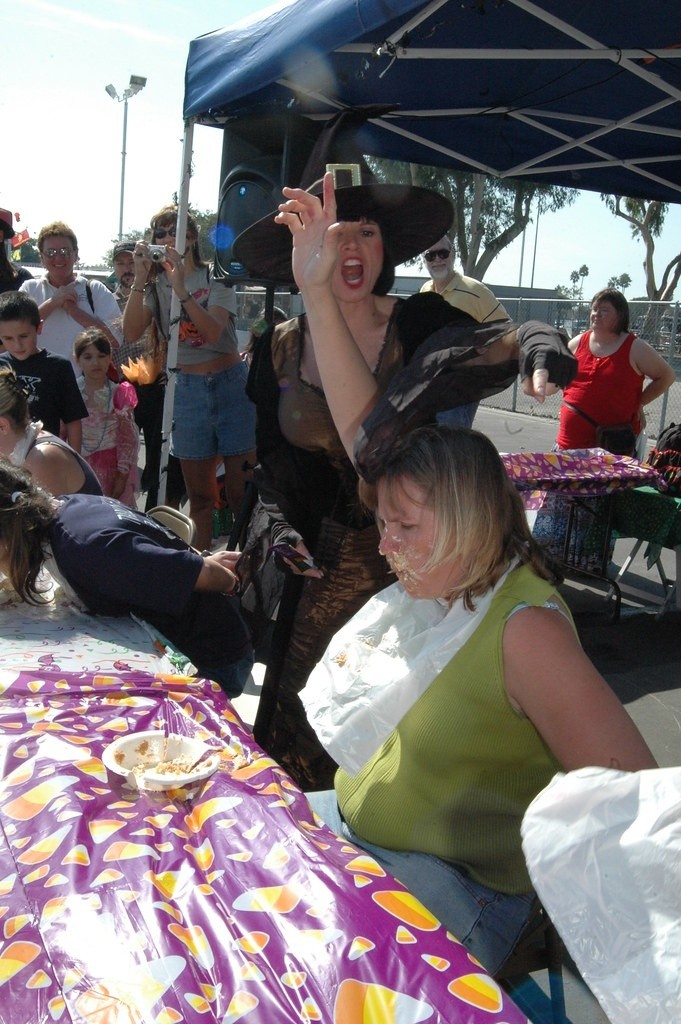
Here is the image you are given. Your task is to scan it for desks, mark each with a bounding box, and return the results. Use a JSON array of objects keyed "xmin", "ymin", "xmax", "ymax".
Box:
[{"xmin": 0, "ymin": 447, "xmax": 681, "ymax": 1024}]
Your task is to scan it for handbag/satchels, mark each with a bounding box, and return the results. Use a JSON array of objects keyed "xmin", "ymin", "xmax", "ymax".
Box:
[{"xmin": 595, "ymin": 424, "xmax": 636, "ymax": 458}]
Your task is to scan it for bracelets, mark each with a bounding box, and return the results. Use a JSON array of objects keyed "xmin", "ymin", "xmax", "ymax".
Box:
[
  {"xmin": 177, "ymin": 290, "xmax": 192, "ymax": 303},
  {"xmin": 220, "ymin": 576, "xmax": 240, "ymax": 598},
  {"xmin": 130, "ymin": 285, "xmax": 146, "ymax": 293}
]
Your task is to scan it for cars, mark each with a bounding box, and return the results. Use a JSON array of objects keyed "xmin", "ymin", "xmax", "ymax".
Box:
[{"xmin": 554, "ymin": 315, "xmax": 681, "ymax": 366}]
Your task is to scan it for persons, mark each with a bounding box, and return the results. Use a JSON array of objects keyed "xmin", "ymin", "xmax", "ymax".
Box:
[
  {"xmin": 274, "ymin": 168, "xmax": 660, "ymax": 976},
  {"xmin": 531, "ymin": 289, "xmax": 678, "ymax": 578},
  {"xmin": 0, "ymin": 206, "xmax": 580, "ymax": 798}
]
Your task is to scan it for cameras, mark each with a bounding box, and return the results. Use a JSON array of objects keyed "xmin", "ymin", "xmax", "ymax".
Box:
[{"xmin": 145, "ymin": 245, "xmax": 169, "ymax": 263}]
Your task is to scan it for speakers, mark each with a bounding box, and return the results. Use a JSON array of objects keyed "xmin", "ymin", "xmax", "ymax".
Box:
[{"xmin": 207, "ymin": 113, "xmax": 319, "ymax": 296}]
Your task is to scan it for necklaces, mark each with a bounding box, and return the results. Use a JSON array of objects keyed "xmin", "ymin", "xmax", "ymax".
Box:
[{"xmin": 83, "ymin": 376, "xmax": 112, "ymax": 453}]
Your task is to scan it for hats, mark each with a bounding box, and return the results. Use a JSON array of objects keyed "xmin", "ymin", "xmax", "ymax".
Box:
[
  {"xmin": 0, "ymin": 208, "xmax": 15, "ymax": 239},
  {"xmin": 231, "ymin": 101, "xmax": 455, "ymax": 281},
  {"xmin": 112, "ymin": 240, "xmax": 135, "ymax": 261}
]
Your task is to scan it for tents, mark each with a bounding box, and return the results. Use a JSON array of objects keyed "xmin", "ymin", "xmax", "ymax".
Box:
[{"xmin": 157, "ymin": 0, "xmax": 681, "ymax": 507}]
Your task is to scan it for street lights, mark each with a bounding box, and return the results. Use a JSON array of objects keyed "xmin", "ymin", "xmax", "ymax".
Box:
[{"xmin": 105, "ymin": 75, "xmax": 147, "ymax": 241}]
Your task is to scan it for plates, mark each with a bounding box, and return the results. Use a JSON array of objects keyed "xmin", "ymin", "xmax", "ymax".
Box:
[{"xmin": 102, "ymin": 730, "xmax": 220, "ymax": 791}]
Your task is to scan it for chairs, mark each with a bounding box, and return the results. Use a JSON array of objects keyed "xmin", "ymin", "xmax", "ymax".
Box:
[
  {"xmin": 226, "ymin": 466, "xmax": 322, "ymax": 746},
  {"xmin": 145, "ymin": 505, "xmax": 195, "ymax": 546},
  {"xmin": 493, "ymin": 896, "xmax": 564, "ymax": 1024}
]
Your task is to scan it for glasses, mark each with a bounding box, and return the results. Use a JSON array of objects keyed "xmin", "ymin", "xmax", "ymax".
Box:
[
  {"xmin": 424, "ymin": 249, "xmax": 451, "ymax": 262},
  {"xmin": 41, "ymin": 247, "xmax": 76, "ymax": 257},
  {"xmin": 154, "ymin": 226, "xmax": 176, "ymax": 239}
]
